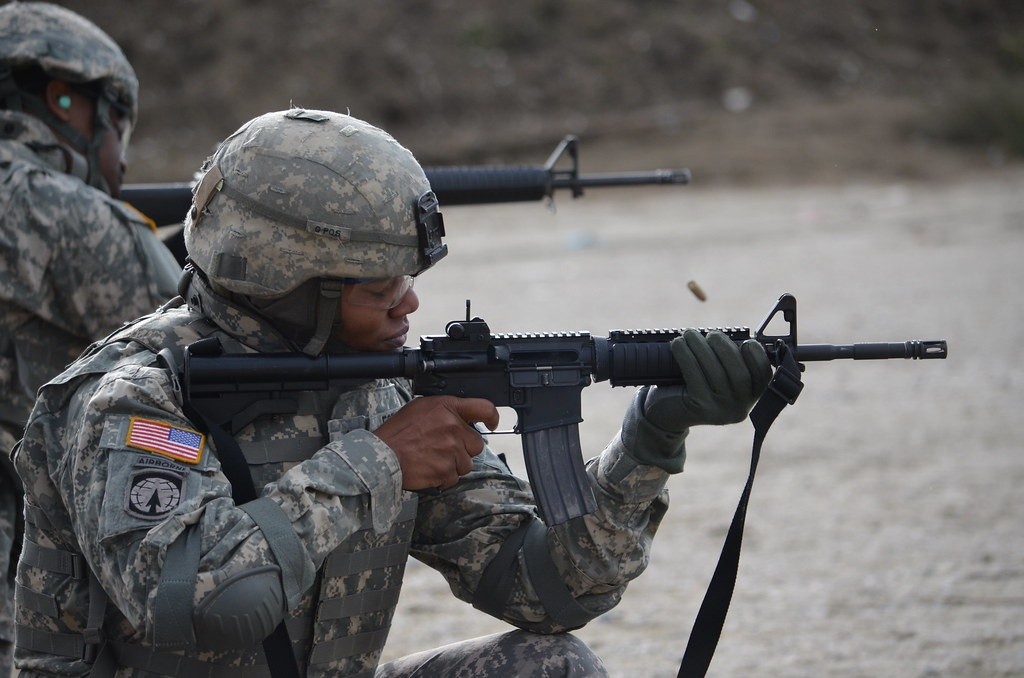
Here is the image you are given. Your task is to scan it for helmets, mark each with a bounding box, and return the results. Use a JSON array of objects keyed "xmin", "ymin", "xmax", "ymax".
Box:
[
  {"xmin": 178, "ymin": 109, "xmax": 449, "ymax": 356},
  {"xmin": 1, "ymin": 1, "xmax": 138, "ymax": 187}
]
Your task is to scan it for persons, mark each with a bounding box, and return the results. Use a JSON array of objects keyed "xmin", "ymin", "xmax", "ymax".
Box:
[
  {"xmin": 1, "ymin": 0, "xmax": 186, "ymax": 677},
  {"xmin": 12, "ymin": 109, "xmax": 773, "ymax": 678}
]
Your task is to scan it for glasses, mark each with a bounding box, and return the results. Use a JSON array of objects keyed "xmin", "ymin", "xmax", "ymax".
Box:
[
  {"xmin": 321, "ymin": 275, "xmax": 414, "ymax": 309},
  {"xmin": 103, "ymin": 116, "xmax": 130, "ymax": 150}
]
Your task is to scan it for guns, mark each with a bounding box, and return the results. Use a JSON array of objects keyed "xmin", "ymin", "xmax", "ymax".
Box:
[
  {"xmin": 117, "ymin": 130, "xmax": 693, "ymax": 243},
  {"xmin": 183, "ymin": 293, "xmax": 950, "ymax": 532}
]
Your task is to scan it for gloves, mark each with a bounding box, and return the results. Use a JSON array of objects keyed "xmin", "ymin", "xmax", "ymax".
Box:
[{"xmin": 621, "ymin": 329, "xmax": 773, "ymax": 474}]
